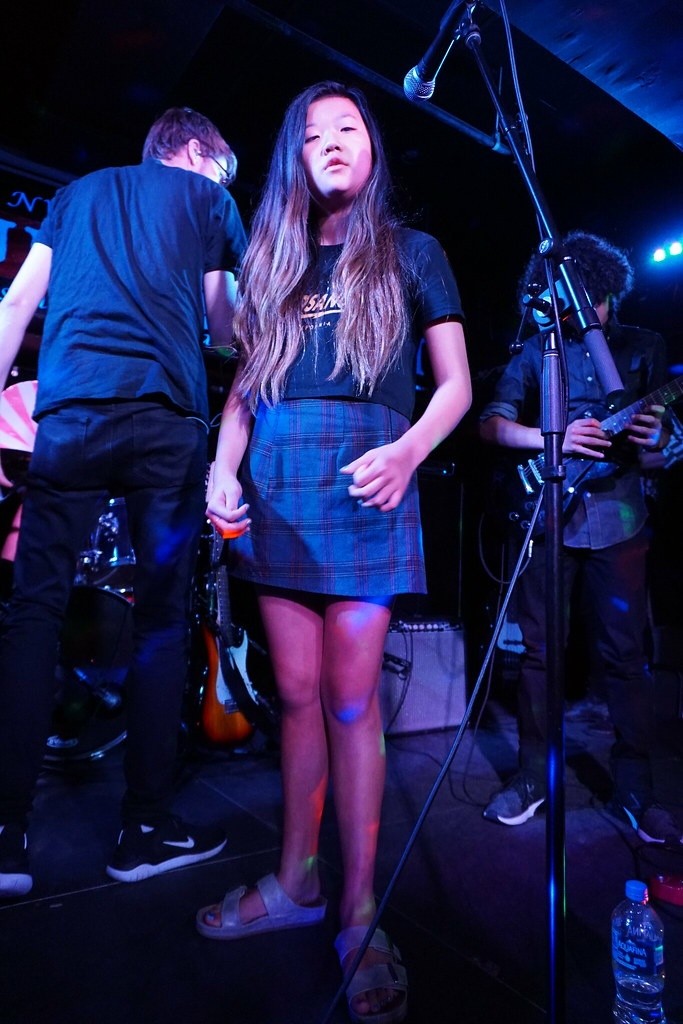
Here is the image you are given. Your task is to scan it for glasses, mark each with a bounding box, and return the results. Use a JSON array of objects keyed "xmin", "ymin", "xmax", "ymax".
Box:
[{"xmin": 209, "ymin": 155, "xmax": 234, "ymax": 187}]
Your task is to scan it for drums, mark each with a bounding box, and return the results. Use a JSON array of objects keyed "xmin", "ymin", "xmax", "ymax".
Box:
[{"xmin": 41, "ymin": 498, "xmax": 136, "ymax": 772}]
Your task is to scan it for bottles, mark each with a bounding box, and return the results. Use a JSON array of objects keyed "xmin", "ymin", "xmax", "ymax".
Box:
[{"xmin": 612, "ymin": 879, "xmax": 667, "ymax": 1024}]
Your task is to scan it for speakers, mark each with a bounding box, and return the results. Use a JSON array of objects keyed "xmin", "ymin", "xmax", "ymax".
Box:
[{"xmin": 374, "ymin": 620, "xmax": 473, "ymax": 735}]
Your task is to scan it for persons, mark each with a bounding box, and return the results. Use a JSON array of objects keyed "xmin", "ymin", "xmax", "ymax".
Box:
[
  {"xmin": 482, "ymin": 236, "xmax": 683, "ymax": 846},
  {"xmin": 0, "ymin": 107, "xmax": 251, "ymax": 894},
  {"xmin": 196, "ymin": 81, "xmax": 472, "ymax": 1024}
]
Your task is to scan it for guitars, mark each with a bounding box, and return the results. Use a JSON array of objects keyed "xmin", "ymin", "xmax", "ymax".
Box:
[
  {"xmin": 190, "ymin": 465, "xmax": 261, "ymax": 748},
  {"xmin": 482, "ymin": 371, "xmax": 683, "ymax": 543}
]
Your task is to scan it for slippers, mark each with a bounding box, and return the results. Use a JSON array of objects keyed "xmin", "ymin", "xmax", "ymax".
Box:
[
  {"xmin": 196, "ymin": 873, "xmax": 328, "ymax": 940},
  {"xmin": 334, "ymin": 926, "xmax": 408, "ymax": 1024}
]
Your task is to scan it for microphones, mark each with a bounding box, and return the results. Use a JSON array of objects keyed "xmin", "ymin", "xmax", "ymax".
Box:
[
  {"xmin": 403, "ymin": 0, "xmax": 478, "ymax": 103},
  {"xmin": 398, "ymin": 661, "xmax": 412, "ymax": 679}
]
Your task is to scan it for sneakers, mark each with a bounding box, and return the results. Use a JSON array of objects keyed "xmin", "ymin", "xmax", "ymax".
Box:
[
  {"xmin": 106, "ymin": 813, "xmax": 231, "ymax": 882},
  {"xmin": 0, "ymin": 823, "xmax": 33, "ymax": 896},
  {"xmin": 606, "ymin": 784, "xmax": 683, "ymax": 843},
  {"xmin": 483, "ymin": 772, "xmax": 546, "ymax": 825}
]
